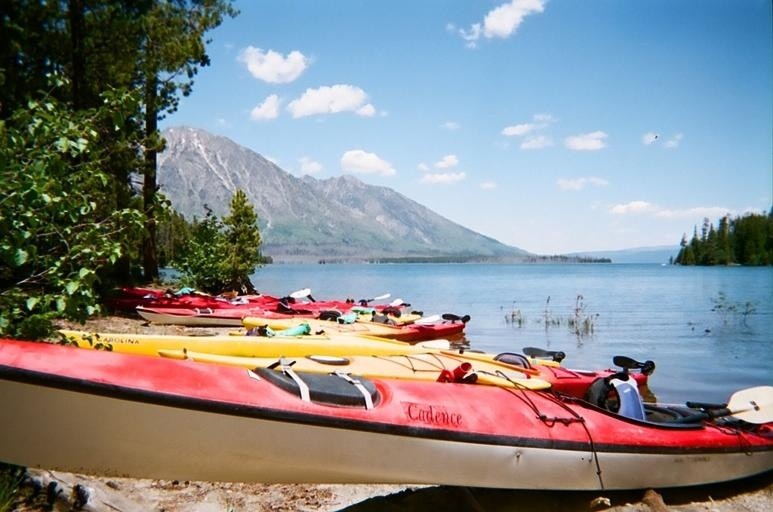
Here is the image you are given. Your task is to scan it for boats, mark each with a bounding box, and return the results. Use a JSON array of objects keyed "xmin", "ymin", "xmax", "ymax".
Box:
[
  {"xmin": 0, "ymin": 339, "xmax": 773, "ymax": 492},
  {"xmin": 52, "ymin": 285, "xmax": 656, "ymax": 401}
]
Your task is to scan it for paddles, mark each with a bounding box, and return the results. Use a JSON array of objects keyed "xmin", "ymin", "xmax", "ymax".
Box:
[
  {"xmin": 160, "ymin": 349, "xmax": 553, "ymax": 390},
  {"xmin": 668, "ymin": 386, "xmax": 773, "ymax": 424},
  {"xmin": 281, "ymin": 288, "xmax": 312, "ymax": 300},
  {"xmin": 367, "ymin": 294, "xmax": 403, "ymax": 312},
  {"xmin": 397, "ymin": 315, "xmax": 440, "ymax": 326}
]
